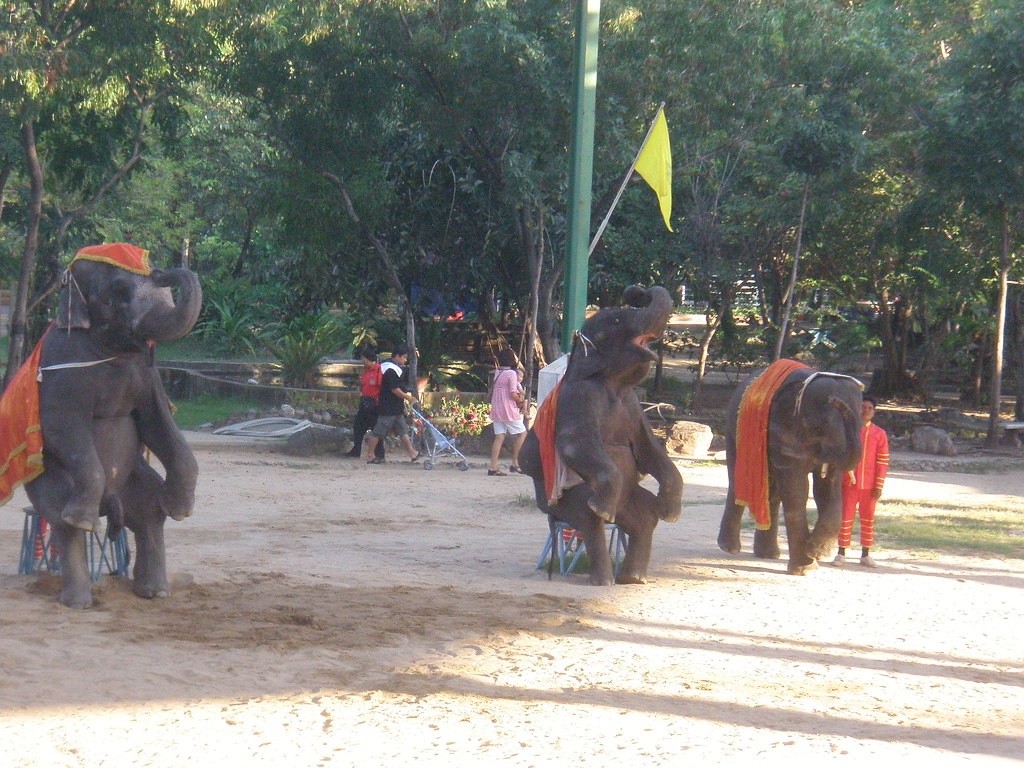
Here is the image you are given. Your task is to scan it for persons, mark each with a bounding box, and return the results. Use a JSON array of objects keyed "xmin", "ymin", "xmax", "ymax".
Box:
[
  {"xmin": 346, "ymin": 348, "xmax": 386, "ymax": 464},
  {"xmin": 832, "ymin": 395, "xmax": 890, "ymax": 568},
  {"xmin": 516, "ymin": 369, "xmax": 532, "ymax": 420},
  {"xmin": 562, "ymin": 527, "xmax": 586, "ymax": 555},
  {"xmin": 487, "ymin": 350, "xmax": 527, "ymax": 475},
  {"xmin": 367, "ymin": 344, "xmax": 427, "ymax": 463},
  {"xmin": 31, "ymin": 510, "xmax": 67, "ymax": 571}
]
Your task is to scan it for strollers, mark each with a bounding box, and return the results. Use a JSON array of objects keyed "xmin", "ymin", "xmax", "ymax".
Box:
[{"xmin": 410, "ymin": 400, "xmax": 468, "ymax": 471}]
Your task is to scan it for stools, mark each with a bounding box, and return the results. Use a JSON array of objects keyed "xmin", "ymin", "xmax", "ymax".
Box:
[
  {"xmin": 17, "ymin": 506, "xmax": 131, "ymax": 580},
  {"xmin": 536, "ymin": 520, "xmax": 628, "ymax": 576}
]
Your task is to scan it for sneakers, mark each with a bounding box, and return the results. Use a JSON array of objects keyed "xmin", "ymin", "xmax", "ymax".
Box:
[
  {"xmin": 832, "ymin": 553, "xmax": 845, "ymax": 566},
  {"xmin": 860, "ymin": 555, "xmax": 879, "ymax": 568}
]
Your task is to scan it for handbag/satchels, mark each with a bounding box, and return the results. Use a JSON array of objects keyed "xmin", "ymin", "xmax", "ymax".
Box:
[{"xmin": 487, "ymin": 389, "xmax": 493, "ymax": 402}]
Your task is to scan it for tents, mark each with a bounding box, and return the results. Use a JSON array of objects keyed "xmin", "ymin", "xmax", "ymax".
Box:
[{"xmin": 537, "ymin": 352, "xmax": 571, "ymax": 411}]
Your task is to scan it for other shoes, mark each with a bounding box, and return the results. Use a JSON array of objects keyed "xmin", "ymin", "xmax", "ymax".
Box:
[
  {"xmin": 509, "ymin": 465, "xmax": 524, "ymax": 475},
  {"xmin": 346, "ymin": 451, "xmax": 361, "ymax": 458},
  {"xmin": 488, "ymin": 468, "xmax": 507, "ymax": 476}
]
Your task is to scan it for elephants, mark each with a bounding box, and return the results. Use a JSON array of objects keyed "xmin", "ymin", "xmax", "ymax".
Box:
[
  {"xmin": 516, "ymin": 282, "xmax": 684, "ymax": 587},
  {"xmin": 0, "ymin": 241, "xmax": 203, "ymax": 612},
  {"xmin": 718, "ymin": 357, "xmax": 867, "ymax": 579}
]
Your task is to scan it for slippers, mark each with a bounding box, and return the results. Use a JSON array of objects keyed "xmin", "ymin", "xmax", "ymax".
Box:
[
  {"xmin": 366, "ymin": 457, "xmax": 387, "ymax": 465},
  {"xmin": 411, "ymin": 452, "xmax": 425, "ymax": 461}
]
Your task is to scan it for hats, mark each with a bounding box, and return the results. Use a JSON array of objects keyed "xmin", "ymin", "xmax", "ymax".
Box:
[{"xmin": 862, "ymin": 396, "xmax": 876, "ymax": 408}]
